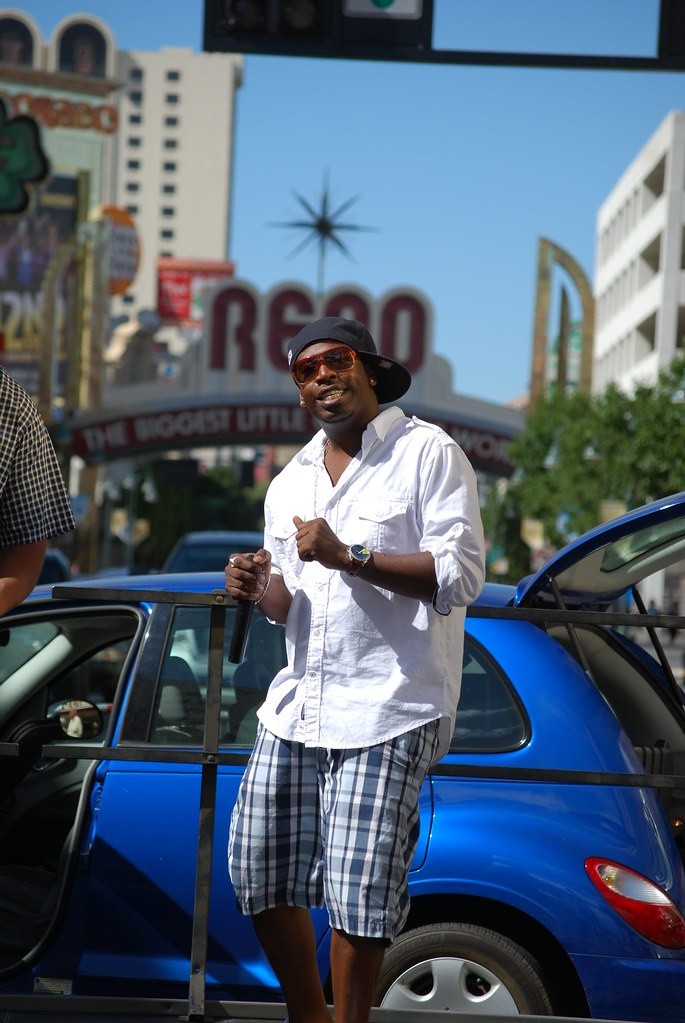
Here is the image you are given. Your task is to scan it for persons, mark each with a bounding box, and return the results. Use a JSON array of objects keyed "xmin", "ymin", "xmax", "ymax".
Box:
[
  {"xmin": 0, "ymin": 365, "xmax": 92, "ymax": 621},
  {"xmin": 0, "ymin": 211, "xmax": 58, "ymax": 296},
  {"xmin": 648, "ymin": 599, "xmax": 680, "ymax": 648},
  {"xmin": 222, "ymin": 318, "xmax": 487, "ymax": 1023}
]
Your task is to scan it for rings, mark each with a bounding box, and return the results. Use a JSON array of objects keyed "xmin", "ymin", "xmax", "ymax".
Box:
[{"xmin": 230, "ymin": 556, "xmax": 237, "ymax": 568}]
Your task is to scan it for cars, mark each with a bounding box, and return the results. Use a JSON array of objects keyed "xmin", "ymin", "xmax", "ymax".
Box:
[
  {"xmin": 0, "ymin": 476, "xmax": 685, "ymax": 1023},
  {"xmin": 154, "ymin": 532, "xmax": 267, "ymax": 575}
]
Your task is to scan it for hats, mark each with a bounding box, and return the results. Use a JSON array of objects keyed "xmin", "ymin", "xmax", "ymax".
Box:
[{"xmin": 286, "ymin": 318, "xmax": 413, "ymax": 404}]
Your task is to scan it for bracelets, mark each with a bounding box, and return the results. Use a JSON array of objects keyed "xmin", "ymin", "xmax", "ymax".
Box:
[{"xmin": 254, "ymin": 575, "xmax": 272, "ymax": 604}]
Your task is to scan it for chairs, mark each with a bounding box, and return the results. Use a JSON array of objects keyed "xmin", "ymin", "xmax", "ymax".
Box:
[
  {"xmin": 229, "ymin": 660, "xmax": 274, "ymax": 745},
  {"xmin": 148, "ymin": 656, "xmax": 205, "ymax": 744}
]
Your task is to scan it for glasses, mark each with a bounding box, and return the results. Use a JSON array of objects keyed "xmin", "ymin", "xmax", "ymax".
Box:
[{"xmin": 291, "ymin": 345, "xmax": 359, "ymax": 386}]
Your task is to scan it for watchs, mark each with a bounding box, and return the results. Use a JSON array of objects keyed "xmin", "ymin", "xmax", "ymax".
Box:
[{"xmin": 347, "ymin": 545, "xmax": 371, "ymax": 577}]
